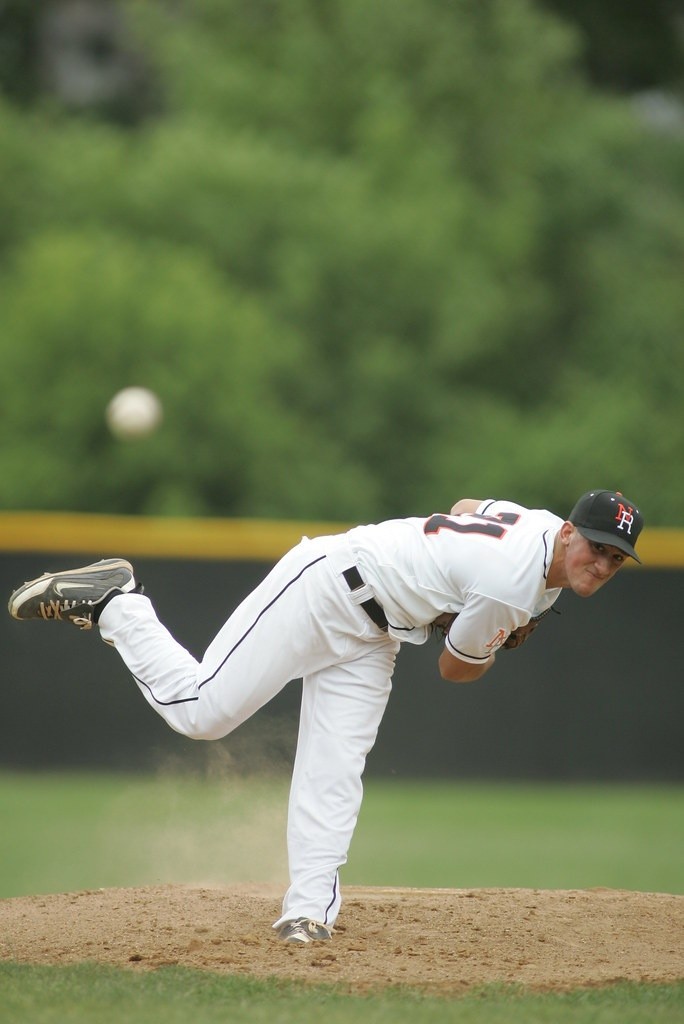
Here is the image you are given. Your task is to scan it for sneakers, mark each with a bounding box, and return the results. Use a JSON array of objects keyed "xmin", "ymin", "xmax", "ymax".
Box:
[
  {"xmin": 278, "ymin": 917, "xmax": 343, "ymax": 942},
  {"xmin": 8, "ymin": 558, "xmax": 145, "ymax": 630}
]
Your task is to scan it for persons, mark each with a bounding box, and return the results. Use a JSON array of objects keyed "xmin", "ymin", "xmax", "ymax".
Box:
[{"xmin": 7, "ymin": 486, "xmax": 643, "ymax": 941}]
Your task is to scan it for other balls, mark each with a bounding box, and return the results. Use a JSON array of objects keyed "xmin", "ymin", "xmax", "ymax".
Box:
[{"xmin": 105, "ymin": 386, "xmax": 164, "ymax": 444}]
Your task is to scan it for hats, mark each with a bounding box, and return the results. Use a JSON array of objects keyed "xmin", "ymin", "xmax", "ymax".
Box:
[{"xmin": 568, "ymin": 488, "xmax": 643, "ymax": 565}]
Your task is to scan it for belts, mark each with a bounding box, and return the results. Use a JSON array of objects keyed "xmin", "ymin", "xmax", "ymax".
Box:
[{"xmin": 343, "ymin": 566, "xmax": 389, "ymax": 632}]
